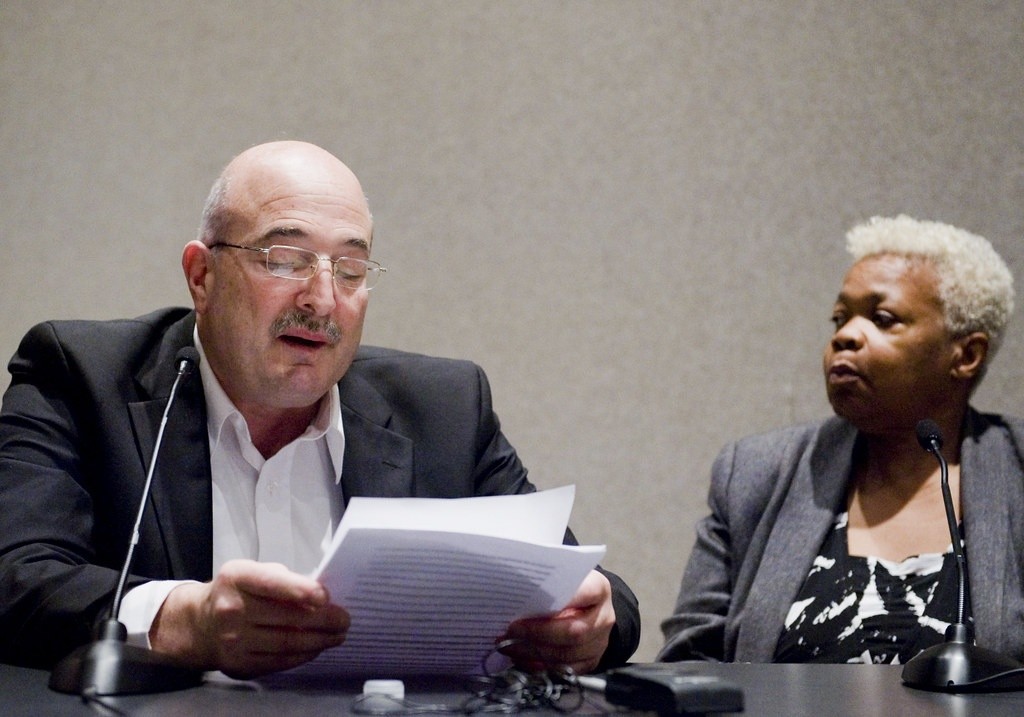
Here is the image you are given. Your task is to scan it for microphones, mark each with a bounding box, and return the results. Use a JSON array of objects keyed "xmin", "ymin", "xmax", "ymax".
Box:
[
  {"xmin": 901, "ymin": 420, "xmax": 1024, "ymax": 693},
  {"xmin": 46, "ymin": 346, "xmax": 202, "ymax": 699}
]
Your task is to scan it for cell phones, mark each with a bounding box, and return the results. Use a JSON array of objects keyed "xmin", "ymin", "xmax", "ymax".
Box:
[{"xmin": 605, "ymin": 664, "xmax": 744, "ymax": 716}]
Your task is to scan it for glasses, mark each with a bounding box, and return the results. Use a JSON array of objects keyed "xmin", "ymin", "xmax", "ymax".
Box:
[{"xmin": 207, "ymin": 242, "xmax": 388, "ymax": 291}]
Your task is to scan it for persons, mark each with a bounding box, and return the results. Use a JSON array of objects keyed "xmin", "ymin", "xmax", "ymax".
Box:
[
  {"xmin": 0, "ymin": 139, "xmax": 642, "ymax": 679},
  {"xmin": 655, "ymin": 213, "xmax": 1024, "ymax": 662}
]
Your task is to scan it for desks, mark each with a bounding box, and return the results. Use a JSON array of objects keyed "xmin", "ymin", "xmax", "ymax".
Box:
[{"xmin": 0, "ymin": 666, "xmax": 1023, "ymax": 717}]
